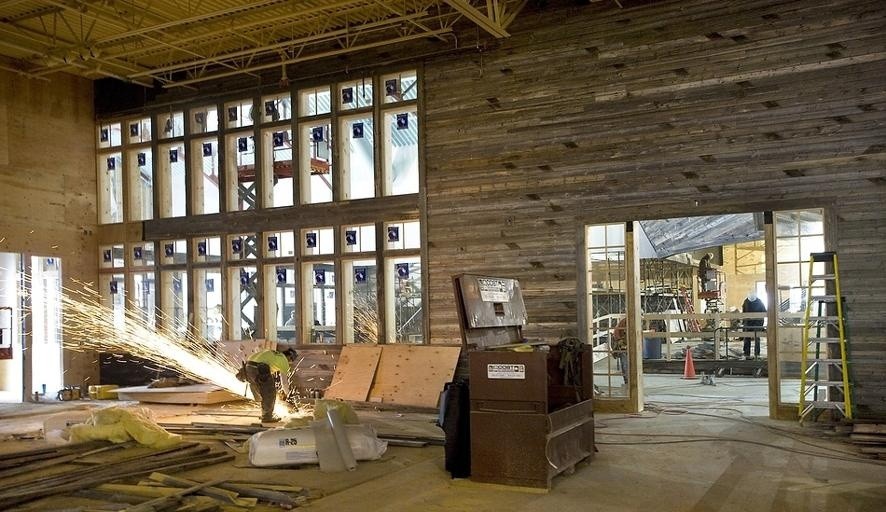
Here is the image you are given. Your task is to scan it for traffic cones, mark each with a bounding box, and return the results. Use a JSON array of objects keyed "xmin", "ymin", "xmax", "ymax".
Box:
[{"xmin": 680, "ymin": 348, "xmax": 699, "ymax": 380}]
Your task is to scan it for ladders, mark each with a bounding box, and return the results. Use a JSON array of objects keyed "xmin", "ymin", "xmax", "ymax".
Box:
[
  {"xmin": 681, "ymin": 287, "xmax": 701, "ymax": 332},
  {"xmin": 812, "ymin": 296, "xmax": 851, "ymax": 421},
  {"xmin": 797, "ymin": 252, "xmax": 852, "ymax": 424}
]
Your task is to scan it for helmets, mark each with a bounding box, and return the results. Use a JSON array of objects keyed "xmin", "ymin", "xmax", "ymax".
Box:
[
  {"xmin": 747, "ymin": 292, "xmax": 756, "ymax": 301},
  {"xmin": 707, "ymin": 253, "xmax": 713, "ymax": 260}
]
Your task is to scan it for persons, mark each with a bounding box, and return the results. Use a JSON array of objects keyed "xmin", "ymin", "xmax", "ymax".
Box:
[
  {"xmin": 741, "ymin": 291, "xmax": 767, "ymax": 359},
  {"xmin": 612, "ymin": 307, "xmax": 644, "ymax": 381},
  {"xmin": 698, "ymin": 253, "xmax": 714, "ymax": 291},
  {"xmin": 235, "ymin": 346, "xmax": 297, "ymax": 424}
]
